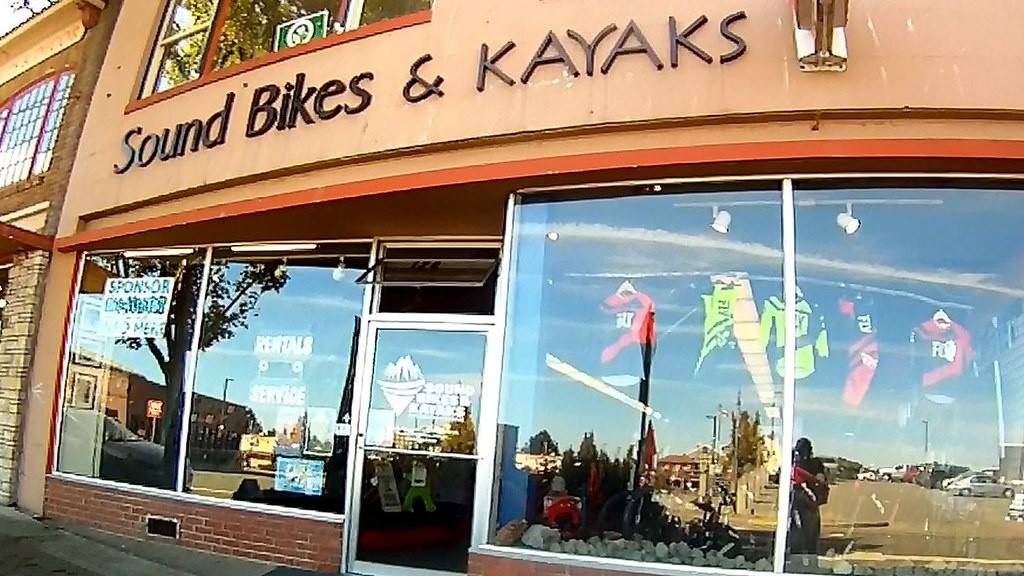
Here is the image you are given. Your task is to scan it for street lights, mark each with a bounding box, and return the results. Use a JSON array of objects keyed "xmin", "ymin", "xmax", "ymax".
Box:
[{"xmin": 223, "ymin": 378, "xmax": 235, "ymax": 401}]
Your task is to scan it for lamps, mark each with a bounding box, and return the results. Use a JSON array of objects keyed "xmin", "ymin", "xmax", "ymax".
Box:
[
  {"xmin": 273, "ymin": 256, "xmax": 287, "ymax": 280},
  {"xmin": 710, "ymin": 204, "xmax": 730, "ymax": 236},
  {"xmin": 332, "ymin": 256, "xmax": 346, "ymax": 282},
  {"xmin": 836, "ymin": 203, "xmax": 860, "ymax": 235}
]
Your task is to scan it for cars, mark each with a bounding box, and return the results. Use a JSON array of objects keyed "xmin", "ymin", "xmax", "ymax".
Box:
[
  {"xmin": 855, "ymin": 461, "xmax": 1015, "ymax": 500},
  {"xmin": 102, "ymin": 415, "xmax": 194, "ymax": 492}
]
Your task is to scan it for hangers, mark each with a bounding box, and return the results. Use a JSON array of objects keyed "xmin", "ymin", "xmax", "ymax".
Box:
[
  {"xmin": 934, "ymin": 308, "xmax": 952, "ymax": 324},
  {"xmin": 618, "ymin": 278, "xmax": 632, "ymax": 294}
]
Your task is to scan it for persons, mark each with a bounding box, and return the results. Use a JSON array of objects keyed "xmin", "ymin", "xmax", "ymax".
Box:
[{"xmin": 785, "ymin": 438, "xmax": 830, "ymax": 555}]
